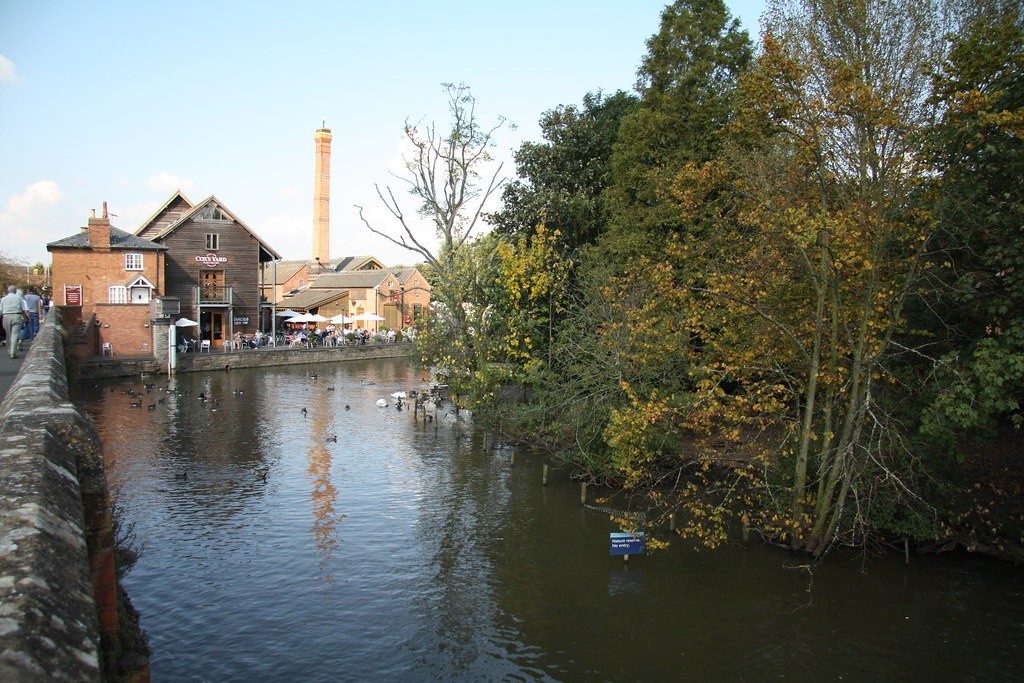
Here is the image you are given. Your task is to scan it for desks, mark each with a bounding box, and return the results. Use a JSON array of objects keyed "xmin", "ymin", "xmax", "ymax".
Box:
[{"xmin": 187, "ymin": 340, "xmax": 197, "ymax": 353}]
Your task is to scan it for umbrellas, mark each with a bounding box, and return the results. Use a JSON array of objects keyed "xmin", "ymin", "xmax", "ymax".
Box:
[
  {"xmin": 174, "ymin": 318, "xmax": 198, "ymax": 328},
  {"xmin": 271, "ymin": 308, "xmax": 303, "ymax": 317},
  {"xmin": 328, "ymin": 314, "xmax": 353, "ymax": 325},
  {"xmin": 284, "ymin": 313, "xmax": 327, "ymax": 330},
  {"xmin": 351, "ymin": 312, "xmax": 386, "ymax": 331}
]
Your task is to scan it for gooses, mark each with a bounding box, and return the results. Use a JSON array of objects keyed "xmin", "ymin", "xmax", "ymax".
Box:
[
  {"xmin": 390, "ymin": 390, "xmax": 406, "ymax": 398},
  {"xmin": 376, "ymin": 398, "xmax": 387, "ymax": 407}
]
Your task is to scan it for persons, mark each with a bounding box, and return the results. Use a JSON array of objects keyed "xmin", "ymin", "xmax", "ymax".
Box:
[
  {"xmin": 173, "ymin": 323, "xmax": 395, "ymax": 353},
  {"xmin": 0, "ymin": 284, "xmax": 49, "ymax": 359}
]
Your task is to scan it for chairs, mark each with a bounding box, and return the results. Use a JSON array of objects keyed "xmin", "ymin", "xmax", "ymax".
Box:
[
  {"xmin": 323, "ymin": 331, "xmax": 343, "ymax": 347},
  {"xmin": 102, "ymin": 342, "xmax": 114, "ymax": 357},
  {"xmin": 284, "ymin": 335, "xmax": 290, "ymax": 345},
  {"xmin": 223, "ymin": 339, "xmax": 232, "ymax": 353},
  {"xmin": 388, "ymin": 335, "xmax": 395, "ymax": 344},
  {"xmin": 267, "ymin": 336, "xmax": 274, "ymax": 347},
  {"xmin": 201, "ymin": 339, "xmax": 211, "ymax": 353},
  {"xmin": 241, "ymin": 342, "xmax": 247, "ymax": 349}
]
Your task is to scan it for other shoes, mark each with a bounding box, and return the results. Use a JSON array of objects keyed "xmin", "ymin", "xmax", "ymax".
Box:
[
  {"xmin": 33, "ymin": 333, "xmax": 37, "ymax": 337},
  {"xmin": 1, "ymin": 341, "xmax": 6, "ymax": 346},
  {"xmin": 11, "ymin": 354, "xmax": 17, "ymax": 358},
  {"xmin": 254, "ymin": 348, "xmax": 258, "ymax": 349},
  {"xmin": 18, "ymin": 339, "xmax": 23, "ymax": 350}
]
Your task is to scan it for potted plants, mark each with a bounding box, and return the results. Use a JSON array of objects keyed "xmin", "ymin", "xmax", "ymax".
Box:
[
  {"xmin": 307, "ymin": 333, "xmax": 318, "ymax": 348},
  {"xmin": 346, "ymin": 332, "xmax": 355, "ymax": 346},
  {"xmin": 395, "ymin": 331, "xmax": 403, "ymax": 343}
]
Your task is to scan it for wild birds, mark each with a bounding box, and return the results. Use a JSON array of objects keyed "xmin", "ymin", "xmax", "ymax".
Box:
[
  {"xmin": 175, "ymin": 472, "xmax": 189, "ymax": 479},
  {"xmin": 93, "ymin": 381, "xmax": 244, "ymax": 413},
  {"xmin": 310, "ymin": 373, "xmax": 318, "ymax": 379},
  {"xmin": 257, "ymin": 473, "xmax": 267, "ymax": 480},
  {"xmin": 360, "ymin": 379, "xmax": 444, "ymax": 420},
  {"xmin": 346, "ymin": 406, "xmax": 350, "ymax": 410},
  {"xmin": 327, "ymin": 436, "xmax": 337, "ymax": 442},
  {"xmin": 327, "ymin": 386, "xmax": 335, "ymax": 390},
  {"xmin": 301, "ymin": 407, "xmax": 308, "ymax": 414}
]
